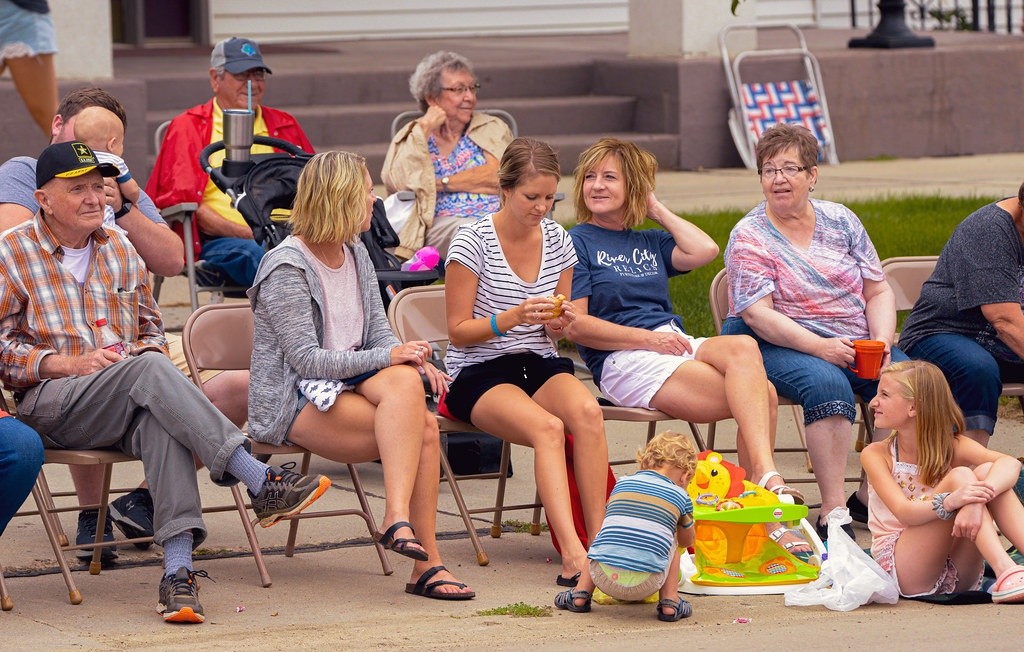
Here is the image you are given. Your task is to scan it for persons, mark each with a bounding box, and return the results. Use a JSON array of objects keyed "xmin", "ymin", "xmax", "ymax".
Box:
[{"xmin": 0, "ymin": 0, "xmax": 1024, "ymax": 624}]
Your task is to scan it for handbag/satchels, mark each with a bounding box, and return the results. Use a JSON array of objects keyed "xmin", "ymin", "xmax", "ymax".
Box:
[
  {"xmin": 445, "ymin": 431, "xmax": 514, "ymax": 478},
  {"xmin": 543, "ymin": 434, "xmax": 617, "ymax": 555},
  {"xmin": 592, "ymin": 586, "xmax": 660, "ymax": 605},
  {"xmin": 784, "ymin": 504, "xmax": 899, "ymax": 612}
]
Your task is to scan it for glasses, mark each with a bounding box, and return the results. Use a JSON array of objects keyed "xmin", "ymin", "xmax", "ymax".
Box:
[
  {"xmin": 759, "ymin": 165, "xmax": 809, "ymax": 179},
  {"xmin": 233, "ymin": 69, "xmax": 267, "ymax": 81},
  {"xmin": 439, "ymin": 83, "xmax": 480, "ymax": 95}
]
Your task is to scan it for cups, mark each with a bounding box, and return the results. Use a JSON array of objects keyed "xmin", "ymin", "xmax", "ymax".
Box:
[
  {"xmin": 222, "ymin": 109, "xmax": 255, "ymax": 162},
  {"xmin": 847, "ymin": 339, "xmax": 886, "ymax": 379}
]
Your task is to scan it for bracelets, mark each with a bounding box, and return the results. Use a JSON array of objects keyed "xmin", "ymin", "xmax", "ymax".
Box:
[
  {"xmin": 491, "ymin": 313, "xmax": 507, "ymax": 337},
  {"xmin": 930, "ymin": 493, "xmax": 957, "ymax": 521},
  {"xmin": 548, "ymin": 324, "xmax": 564, "ymax": 331}
]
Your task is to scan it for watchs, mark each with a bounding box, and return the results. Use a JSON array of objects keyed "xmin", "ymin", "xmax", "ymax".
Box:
[
  {"xmin": 440, "ymin": 175, "xmax": 451, "ymax": 192},
  {"xmin": 114, "ymin": 198, "xmax": 134, "ymax": 219}
]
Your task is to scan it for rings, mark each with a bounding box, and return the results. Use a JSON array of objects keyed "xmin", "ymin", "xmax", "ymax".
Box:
[
  {"xmin": 674, "ymin": 349, "xmax": 679, "ymax": 354},
  {"xmin": 438, "ymin": 370, "xmax": 441, "ymax": 372},
  {"xmin": 415, "ymin": 350, "xmax": 419, "ymax": 355},
  {"xmin": 535, "ymin": 314, "xmax": 538, "ymax": 319},
  {"xmin": 419, "ymin": 346, "xmax": 424, "ymax": 351}
]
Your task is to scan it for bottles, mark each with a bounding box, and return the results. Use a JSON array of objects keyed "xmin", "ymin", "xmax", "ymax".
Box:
[
  {"xmin": 95, "ymin": 317, "xmax": 129, "ymax": 363},
  {"xmin": 400, "ymin": 246, "xmax": 440, "ymax": 272}
]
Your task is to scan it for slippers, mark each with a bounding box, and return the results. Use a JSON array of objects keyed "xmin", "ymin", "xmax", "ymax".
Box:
[
  {"xmin": 405, "ymin": 564, "xmax": 475, "ymax": 599},
  {"xmin": 373, "ymin": 521, "xmax": 429, "ymax": 562},
  {"xmin": 556, "ymin": 572, "xmax": 583, "ymax": 587}
]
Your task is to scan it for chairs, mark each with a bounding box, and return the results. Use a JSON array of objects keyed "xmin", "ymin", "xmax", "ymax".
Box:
[
  {"xmin": 145, "ymin": 108, "xmax": 568, "ymax": 311},
  {"xmin": 708, "ymin": 259, "xmax": 872, "ymax": 517},
  {"xmin": 389, "ymin": 269, "xmax": 567, "ymax": 582},
  {"xmin": 176, "ymin": 289, "xmax": 411, "ymax": 587},
  {"xmin": 874, "ymin": 243, "xmax": 1024, "ymax": 479},
  {"xmin": 0, "ymin": 378, "xmax": 185, "ymax": 609},
  {"xmin": 592, "ymin": 363, "xmax": 701, "ymax": 538}
]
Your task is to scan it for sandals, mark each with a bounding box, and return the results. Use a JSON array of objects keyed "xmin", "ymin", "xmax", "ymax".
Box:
[
  {"xmin": 767, "ymin": 525, "xmax": 814, "ymax": 553},
  {"xmin": 554, "ymin": 587, "xmax": 592, "ymax": 613},
  {"xmin": 657, "ymin": 594, "xmax": 693, "ymax": 622},
  {"xmin": 756, "ymin": 471, "xmax": 805, "ymax": 506},
  {"xmin": 991, "ymin": 565, "xmax": 1024, "ymax": 604}
]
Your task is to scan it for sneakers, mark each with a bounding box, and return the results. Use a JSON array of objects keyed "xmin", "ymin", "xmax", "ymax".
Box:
[
  {"xmin": 75, "ymin": 505, "xmax": 119, "ymax": 560},
  {"xmin": 155, "ymin": 566, "xmax": 217, "ymax": 624},
  {"xmin": 246, "ymin": 460, "xmax": 331, "ymax": 529},
  {"xmin": 108, "ymin": 492, "xmax": 156, "ymax": 549}
]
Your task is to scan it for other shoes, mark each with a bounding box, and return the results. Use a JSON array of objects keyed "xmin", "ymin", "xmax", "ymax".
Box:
[
  {"xmin": 845, "ymin": 491, "xmax": 869, "ymax": 530},
  {"xmin": 814, "ymin": 512, "xmax": 856, "ymax": 541}
]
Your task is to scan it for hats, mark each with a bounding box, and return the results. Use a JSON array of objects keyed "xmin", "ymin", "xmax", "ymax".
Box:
[
  {"xmin": 211, "ymin": 36, "xmax": 272, "ymax": 74},
  {"xmin": 35, "ymin": 140, "xmax": 121, "ymax": 190}
]
